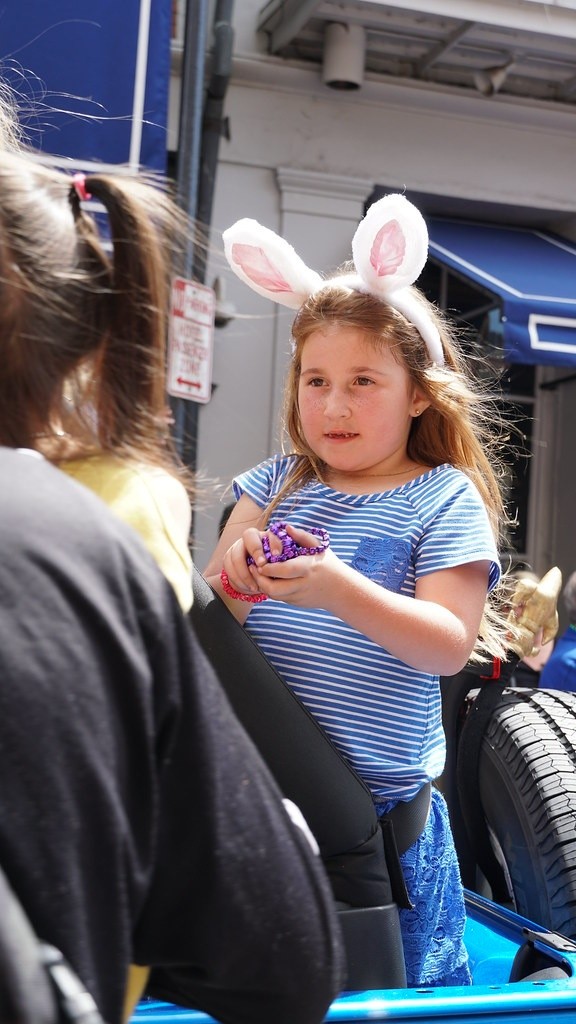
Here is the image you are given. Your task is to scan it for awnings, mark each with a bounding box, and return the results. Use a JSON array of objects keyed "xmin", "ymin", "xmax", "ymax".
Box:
[{"xmin": 423, "ymin": 213, "xmax": 576, "ymax": 368}]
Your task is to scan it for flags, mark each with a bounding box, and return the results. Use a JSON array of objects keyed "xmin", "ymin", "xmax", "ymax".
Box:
[{"xmin": 0, "ymin": 1, "xmax": 169, "ymax": 251}]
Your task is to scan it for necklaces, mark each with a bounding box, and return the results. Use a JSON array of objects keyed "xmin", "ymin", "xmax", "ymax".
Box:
[
  {"xmin": 247, "ymin": 522, "xmax": 334, "ymax": 566},
  {"xmin": 351, "ymin": 466, "xmax": 422, "ymax": 479}
]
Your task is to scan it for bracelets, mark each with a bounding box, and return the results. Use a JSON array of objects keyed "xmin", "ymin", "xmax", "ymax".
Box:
[{"xmin": 220, "ymin": 571, "xmax": 267, "ymax": 603}]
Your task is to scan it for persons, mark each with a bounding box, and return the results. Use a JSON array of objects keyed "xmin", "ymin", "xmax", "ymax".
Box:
[{"xmin": 0, "ymin": 84, "xmax": 576, "ymax": 1024}]
